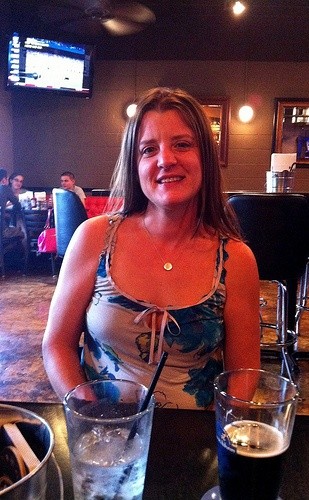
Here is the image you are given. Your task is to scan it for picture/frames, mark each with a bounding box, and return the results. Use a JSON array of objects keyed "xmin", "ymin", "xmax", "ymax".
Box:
[
  {"xmin": 270, "ymin": 96, "xmax": 309, "ymax": 168},
  {"xmin": 194, "ymin": 95, "xmax": 230, "ymax": 166}
]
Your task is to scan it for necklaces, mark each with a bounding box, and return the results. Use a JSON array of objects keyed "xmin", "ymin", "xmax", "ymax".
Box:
[{"xmin": 142, "ymin": 213, "xmax": 200, "ymax": 270}]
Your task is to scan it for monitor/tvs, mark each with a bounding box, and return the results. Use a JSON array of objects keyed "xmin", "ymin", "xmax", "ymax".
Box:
[{"xmin": 4, "ymin": 32, "xmax": 95, "ymax": 99}]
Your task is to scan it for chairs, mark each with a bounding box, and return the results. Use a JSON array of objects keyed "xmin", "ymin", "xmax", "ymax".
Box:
[{"xmin": 22, "ymin": 206, "xmax": 60, "ymax": 277}]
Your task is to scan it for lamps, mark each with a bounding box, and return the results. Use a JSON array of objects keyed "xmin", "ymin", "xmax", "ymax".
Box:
[
  {"xmin": 237, "ymin": 57, "xmax": 256, "ymax": 124},
  {"xmin": 122, "ymin": 64, "xmax": 139, "ymax": 121}
]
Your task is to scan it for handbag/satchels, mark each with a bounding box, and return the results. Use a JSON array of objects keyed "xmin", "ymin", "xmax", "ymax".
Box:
[{"xmin": 38, "ymin": 208, "xmax": 57, "ymax": 254}]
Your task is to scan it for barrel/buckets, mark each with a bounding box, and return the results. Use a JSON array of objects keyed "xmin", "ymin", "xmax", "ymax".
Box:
[
  {"xmin": 265, "ymin": 170, "xmax": 295, "ymax": 193},
  {"xmin": 0, "ymin": 404, "xmax": 64, "ymax": 500}
]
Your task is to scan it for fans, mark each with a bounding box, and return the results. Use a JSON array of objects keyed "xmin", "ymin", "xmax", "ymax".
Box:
[{"xmin": 39, "ymin": 0, "xmax": 155, "ymax": 37}]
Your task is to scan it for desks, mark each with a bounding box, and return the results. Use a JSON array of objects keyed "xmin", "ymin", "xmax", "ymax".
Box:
[{"xmin": 0, "ymin": 399, "xmax": 309, "ymax": 500}]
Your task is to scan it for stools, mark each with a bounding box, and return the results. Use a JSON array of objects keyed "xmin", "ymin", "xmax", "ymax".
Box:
[
  {"xmin": 53, "ymin": 187, "xmax": 87, "ymax": 254},
  {"xmin": 226, "ymin": 193, "xmax": 309, "ymax": 376}
]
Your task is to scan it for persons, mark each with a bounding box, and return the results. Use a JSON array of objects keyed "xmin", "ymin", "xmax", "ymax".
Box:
[
  {"xmin": 6, "ymin": 171, "xmax": 33, "ymax": 209},
  {"xmin": 0, "ymin": 169, "xmax": 33, "ymax": 273},
  {"xmin": 42, "ymin": 87, "xmax": 261, "ymax": 410},
  {"xmin": 60, "ymin": 171, "xmax": 86, "ymax": 207}
]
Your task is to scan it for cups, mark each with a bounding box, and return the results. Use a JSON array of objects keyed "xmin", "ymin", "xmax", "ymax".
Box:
[
  {"xmin": 23, "ymin": 199, "xmax": 31, "ymax": 210},
  {"xmin": 62, "ymin": 379, "xmax": 155, "ymax": 500},
  {"xmin": 31, "ymin": 198, "xmax": 37, "ymax": 210},
  {"xmin": 212, "ymin": 367, "xmax": 299, "ymax": 500}
]
